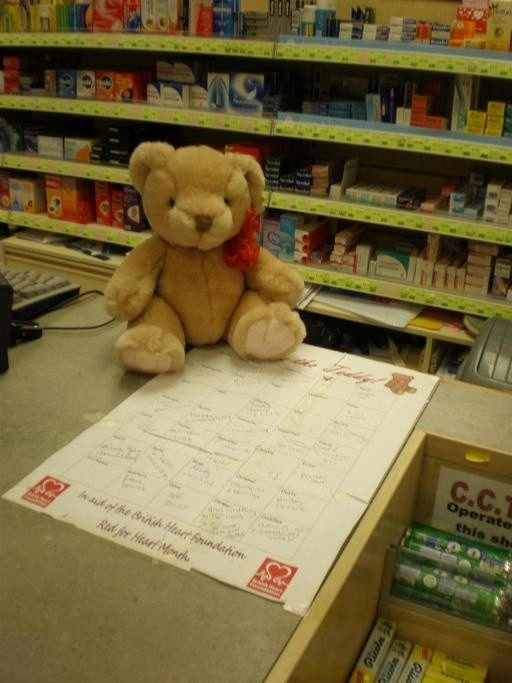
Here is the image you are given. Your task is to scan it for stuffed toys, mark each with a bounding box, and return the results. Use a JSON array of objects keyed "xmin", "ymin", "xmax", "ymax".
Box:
[{"xmin": 103, "ymin": 141, "xmax": 307, "ymax": 374}]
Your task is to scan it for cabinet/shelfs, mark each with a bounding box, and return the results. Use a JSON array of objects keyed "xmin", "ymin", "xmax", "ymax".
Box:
[{"xmin": 0, "ymin": 1, "xmax": 512, "ymax": 381}]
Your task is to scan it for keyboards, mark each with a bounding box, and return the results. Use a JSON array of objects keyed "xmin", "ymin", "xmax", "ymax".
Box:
[{"xmin": 0, "ymin": 265, "xmax": 81, "ymax": 325}]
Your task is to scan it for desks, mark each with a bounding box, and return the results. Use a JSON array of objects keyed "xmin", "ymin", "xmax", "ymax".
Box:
[{"xmin": 0, "ymin": 288, "xmax": 441, "ymax": 683}]
